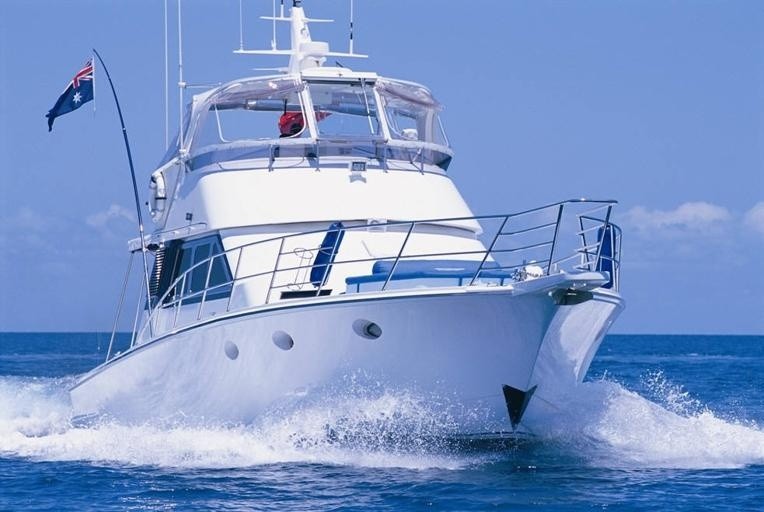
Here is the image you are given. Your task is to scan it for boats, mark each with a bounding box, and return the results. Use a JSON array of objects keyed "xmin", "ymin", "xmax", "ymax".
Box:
[{"xmin": 50, "ymin": 2, "xmax": 626, "ymax": 453}]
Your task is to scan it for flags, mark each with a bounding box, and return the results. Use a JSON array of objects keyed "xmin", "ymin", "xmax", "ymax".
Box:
[{"xmin": 45, "ymin": 57, "xmax": 93, "ymax": 132}]
